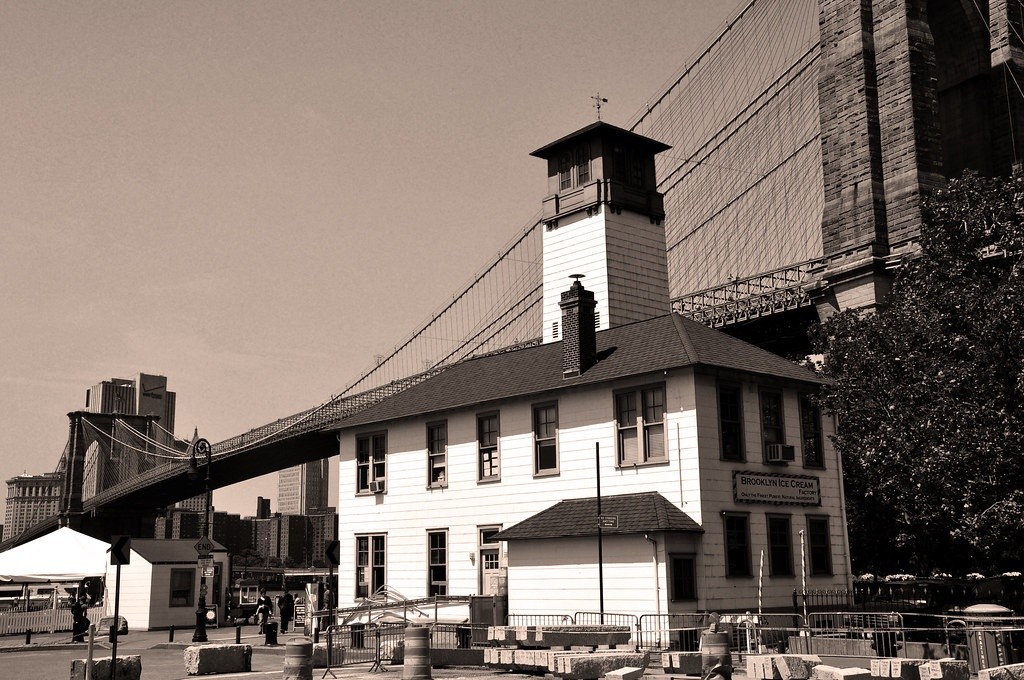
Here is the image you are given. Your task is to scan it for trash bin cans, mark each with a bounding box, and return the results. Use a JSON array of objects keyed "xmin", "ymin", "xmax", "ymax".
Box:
[
  {"xmin": 870, "ymin": 632, "xmax": 902, "ymax": 657},
  {"xmin": 679, "ymin": 627, "xmax": 698, "ymax": 651},
  {"xmin": 963, "ymin": 604, "xmax": 1015, "ymax": 674},
  {"xmin": 349, "ymin": 623, "xmax": 365, "ymax": 649},
  {"xmin": 264, "ymin": 622, "xmax": 279, "ymax": 647},
  {"xmin": 455, "ymin": 626, "xmax": 473, "ymax": 648}
]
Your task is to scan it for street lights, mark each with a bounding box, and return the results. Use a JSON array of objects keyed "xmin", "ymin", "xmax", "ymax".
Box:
[{"xmin": 186, "ymin": 438, "xmax": 215, "ymax": 642}]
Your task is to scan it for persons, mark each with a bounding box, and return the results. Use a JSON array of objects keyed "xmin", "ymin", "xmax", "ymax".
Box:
[
  {"xmin": 71, "ymin": 596, "xmax": 90, "ymax": 636},
  {"xmin": 319, "ymin": 583, "xmax": 335, "ymax": 631},
  {"xmin": 256, "ymin": 588, "xmax": 273, "ymax": 634},
  {"xmin": 278, "ymin": 588, "xmax": 294, "ymax": 633}
]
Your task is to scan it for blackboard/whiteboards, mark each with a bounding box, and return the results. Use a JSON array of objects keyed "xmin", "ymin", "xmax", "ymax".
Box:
[{"xmin": 293, "ymin": 605, "xmax": 305, "ymax": 628}]
